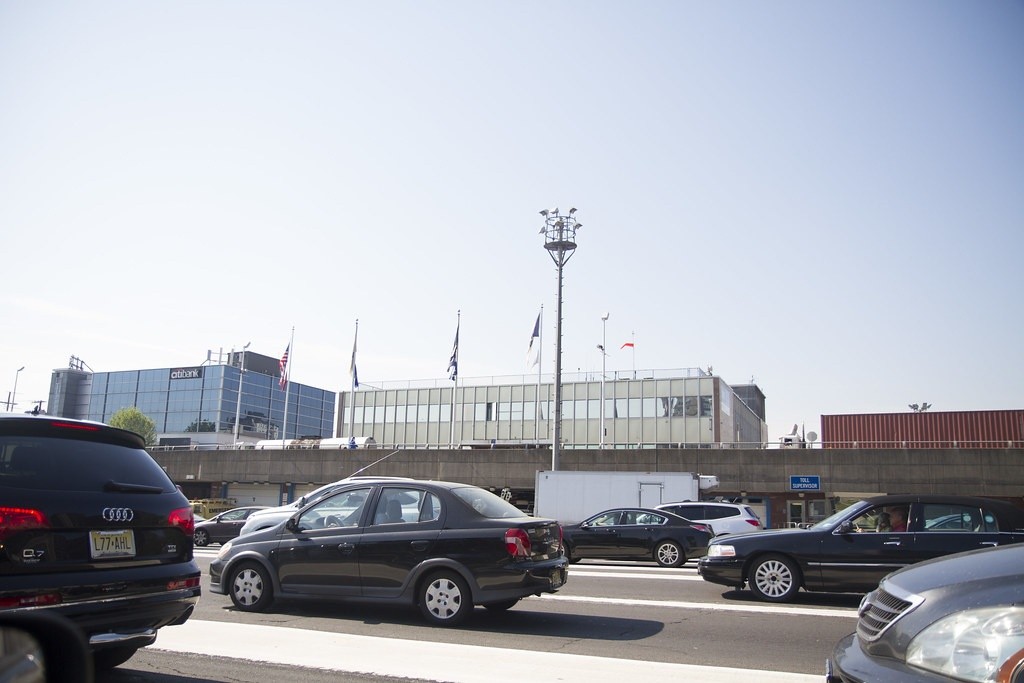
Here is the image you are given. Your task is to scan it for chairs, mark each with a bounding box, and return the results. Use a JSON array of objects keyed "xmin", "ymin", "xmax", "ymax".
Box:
[{"xmin": 383, "ymin": 501, "xmax": 405, "ymax": 524}]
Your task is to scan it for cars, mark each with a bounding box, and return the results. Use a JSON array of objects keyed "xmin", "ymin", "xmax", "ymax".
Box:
[
  {"xmin": 208, "ymin": 477, "xmax": 569, "ymax": 630},
  {"xmin": 239, "ymin": 473, "xmax": 440, "ymax": 537},
  {"xmin": 560, "ymin": 508, "xmax": 716, "ymax": 568},
  {"xmin": 697, "ymin": 490, "xmax": 1024, "ymax": 604},
  {"xmin": 192, "ymin": 505, "xmax": 275, "ymax": 548}
]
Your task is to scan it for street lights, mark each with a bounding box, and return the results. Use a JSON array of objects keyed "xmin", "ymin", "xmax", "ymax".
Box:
[
  {"xmin": 536, "ymin": 206, "xmax": 583, "ymax": 471},
  {"xmin": 233, "ymin": 340, "xmax": 251, "ymax": 451},
  {"xmin": 11, "ymin": 367, "xmax": 25, "ymax": 413},
  {"xmin": 598, "ymin": 311, "xmax": 611, "ymax": 449}
]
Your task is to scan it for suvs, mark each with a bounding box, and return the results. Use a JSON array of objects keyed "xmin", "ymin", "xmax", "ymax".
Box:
[
  {"xmin": 0, "ymin": 411, "xmax": 202, "ymax": 683},
  {"xmin": 634, "ymin": 499, "xmax": 763, "ymax": 538},
  {"xmin": 824, "ymin": 542, "xmax": 1023, "ymax": 683}
]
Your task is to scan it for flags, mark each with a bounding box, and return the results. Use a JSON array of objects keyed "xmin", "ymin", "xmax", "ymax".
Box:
[
  {"xmin": 526, "ymin": 312, "xmax": 541, "ymax": 369},
  {"xmin": 446, "ymin": 325, "xmax": 459, "ymax": 381},
  {"xmin": 279, "ymin": 343, "xmax": 289, "ymax": 391},
  {"xmin": 349, "ymin": 332, "xmax": 359, "ymax": 388}
]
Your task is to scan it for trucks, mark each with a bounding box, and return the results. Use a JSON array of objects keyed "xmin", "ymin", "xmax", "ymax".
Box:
[{"xmin": 534, "ymin": 470, "xmax": 733, "ymax": 528}]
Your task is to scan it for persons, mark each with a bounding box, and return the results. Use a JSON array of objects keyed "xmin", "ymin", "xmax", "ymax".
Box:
[
  {"xmin": 859, "ymin": 509, "xmax": 907, "ymax": 532},
  {"xmin": 351, "ymin": 437, "xmax": 359, "ymax": 449},
  {"xmin": 373, "ymin": 494, "xmax": 390, "ymax": 525}
]
